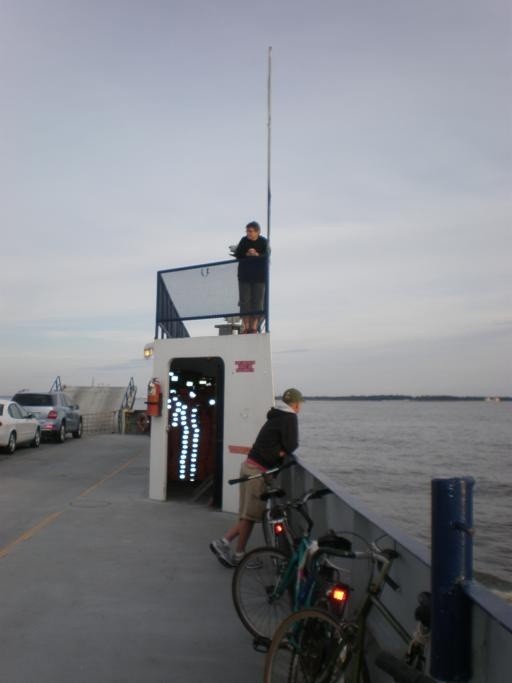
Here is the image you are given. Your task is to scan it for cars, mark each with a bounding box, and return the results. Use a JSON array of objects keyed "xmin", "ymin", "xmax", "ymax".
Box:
[{"xmin": 0, "ymin": 397, "xmax": 43, "ymax": 452}]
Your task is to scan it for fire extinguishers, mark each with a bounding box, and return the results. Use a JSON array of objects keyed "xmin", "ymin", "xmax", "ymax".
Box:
[{"xmin": 146, "ymin": 378, "xmax": 160, "ymax": 415}]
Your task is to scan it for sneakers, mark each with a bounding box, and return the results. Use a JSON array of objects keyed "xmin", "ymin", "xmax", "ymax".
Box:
[
  {"xmin": 209, "ymin": 537, "xmax": 232, "ymax": 569},
  {"xmin": 250, "ymin": 328, "xmax": 258, "ymax": 334},
  {"xmin": 232, "ymin": 551, "xmax": 267, "ymax": 569},
  {"xmin": 240, "ymin": 328, "xmax": 248, "ymax": 334}
]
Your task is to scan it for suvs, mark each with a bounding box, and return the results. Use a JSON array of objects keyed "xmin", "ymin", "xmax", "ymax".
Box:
[{"xmin": 10, "ymin": 386, "xmax": 88, "ymax": 443}]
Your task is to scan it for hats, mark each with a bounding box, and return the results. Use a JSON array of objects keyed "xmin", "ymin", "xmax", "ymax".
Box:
[{"xmin": 282, "ymin": 388, "xmax": 305, "ymax": 403}]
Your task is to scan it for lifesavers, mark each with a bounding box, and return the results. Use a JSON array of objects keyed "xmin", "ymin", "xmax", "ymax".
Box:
[{"xmin": 136, "ymin": 414, "xmax": 150, "ymax": 433}]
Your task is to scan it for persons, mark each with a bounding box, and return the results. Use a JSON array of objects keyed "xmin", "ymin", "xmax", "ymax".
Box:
[
  {"xmin": 235, "ymin": 222, "xmax": 270, "ymax": 334},
  {"xmin": 210, "ymin": 389, "xmax": 303, "ymax": 568}
]
